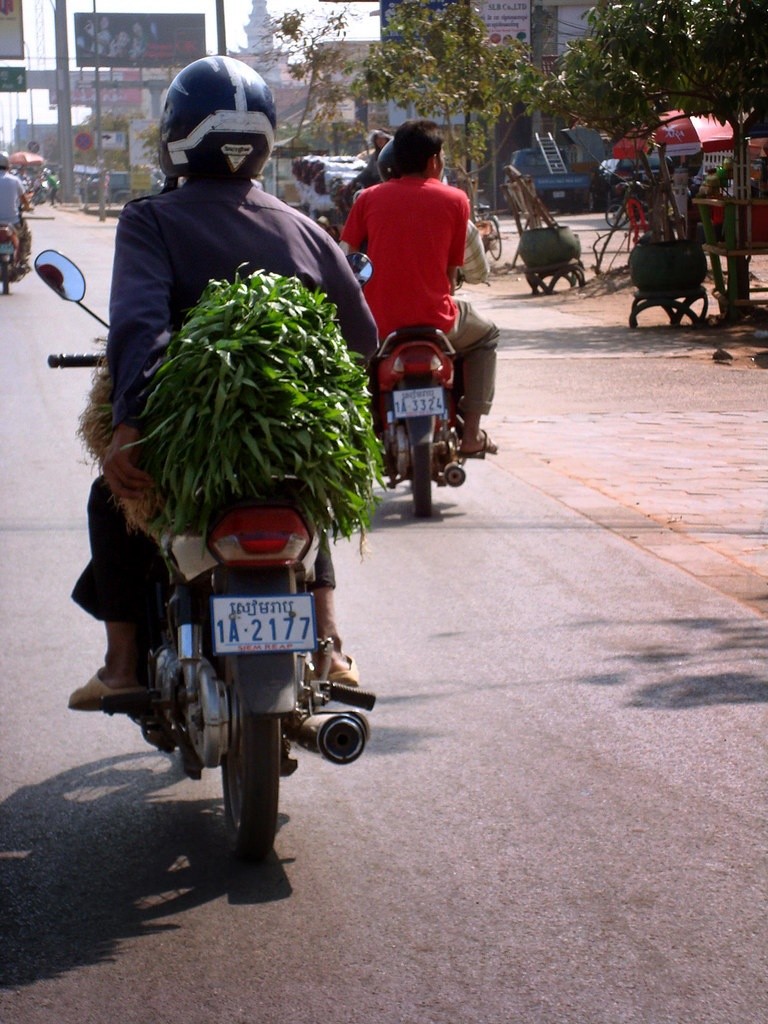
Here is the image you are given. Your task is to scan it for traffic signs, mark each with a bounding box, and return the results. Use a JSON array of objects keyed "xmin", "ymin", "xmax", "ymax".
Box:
[{"xmin": 0, "ymin": 67, "xmax": 27, "ymax": 92}]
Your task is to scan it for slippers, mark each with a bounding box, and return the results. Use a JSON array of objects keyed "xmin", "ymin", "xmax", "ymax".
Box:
[
  {"xmin": 459, "ymin": 429, "xmax": 498, "ymax": 457},
  {"xmin": 325, "ymin": 651, "xmax": 360, "ymax": 689},
  {"xmin": 67, "ymin": 666, "xmax": 145, "ymax": 711}
]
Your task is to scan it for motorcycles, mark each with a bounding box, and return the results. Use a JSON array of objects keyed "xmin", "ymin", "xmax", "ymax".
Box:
[
  {"xmin": 0, "ymin": 180, "xmax": 47, "ymax": 294},
  {"xmin": 36, "ymin": 249, "xmax": 375, "ymax": 858},
  {"xmin": 317, "ymin": 216, "xmax": 491, "ymax": 519}
]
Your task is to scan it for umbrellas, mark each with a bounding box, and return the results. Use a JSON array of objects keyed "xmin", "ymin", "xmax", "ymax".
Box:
[
  {"xmin": 613, "ymin": 108, "xmax": 735, "ymax": 160},
  {"xmin": 9, "ymin": 151, "xmax": 43, "ymax": 165}
]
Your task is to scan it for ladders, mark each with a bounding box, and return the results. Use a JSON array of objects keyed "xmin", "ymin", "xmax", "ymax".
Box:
[{"xmin": 535, "ymin": 132, "xmax": 569, "ymax": 174}]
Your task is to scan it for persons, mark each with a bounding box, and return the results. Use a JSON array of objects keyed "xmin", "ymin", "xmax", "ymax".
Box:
[
  {"xmin": 340, "ymin": 119, "xmax": 500, "ymax": 457},
  {"xmin": 67, "ymin": 53, "xmax": 380, "ymax": 713},
  {"xmin": 0, "ymin": 151, "xmax": 63, "ymax": 273}
]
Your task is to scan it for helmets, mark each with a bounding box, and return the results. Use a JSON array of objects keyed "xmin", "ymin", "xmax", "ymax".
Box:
[
  {"xmin": 370, "ymin": 130, "xmax": 391, "ymax": 152},
  {"xmin": 157, "ymin": 55, "xmax": 276, "ymax": 180},
  {"xmin": 0, "ymin": 151, "xmax": 10, "ymax": 166},
  {"xmin": 376, "ymin": 137, "xmax": 401, "ymax": 182},
  {"xmin": 36, "ymin": 265, "xmax": 64, "ymax": 292}
]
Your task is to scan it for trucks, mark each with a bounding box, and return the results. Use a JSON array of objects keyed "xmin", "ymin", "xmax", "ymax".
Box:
[{"xmin": 504, "ymin": 148, "xmax": 592, "ymax": 211}]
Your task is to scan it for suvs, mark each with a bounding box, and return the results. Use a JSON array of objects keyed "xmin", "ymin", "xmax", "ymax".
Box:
[
  {"xmin": 600, "ymin": 155, "xmax": 667, "ymax": 201},
  {"xmin": 79, "ymin": 172, "xmax": 165, "ymax": 204}
]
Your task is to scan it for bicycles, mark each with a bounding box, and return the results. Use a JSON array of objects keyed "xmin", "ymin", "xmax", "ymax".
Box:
[{"xmin": 475, "ymin": 204, "xmax": 509, "ymax": 260}]
